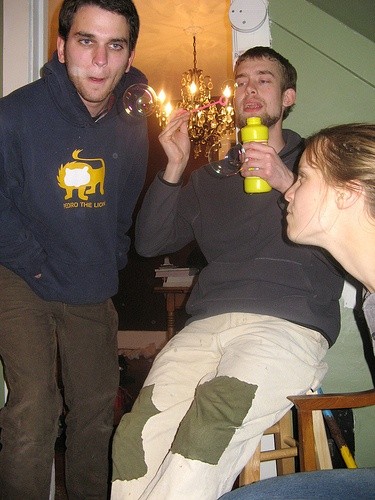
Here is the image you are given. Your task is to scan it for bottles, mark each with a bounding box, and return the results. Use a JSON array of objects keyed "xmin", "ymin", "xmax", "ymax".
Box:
[{"xmin": 242, "ymin": 116, "xmax": 272, "ymax": 194}]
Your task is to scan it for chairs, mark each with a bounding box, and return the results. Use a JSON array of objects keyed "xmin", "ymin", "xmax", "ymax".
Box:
[{"xmin": 286, "ymin": 387, "xmax": 375, "ymax": 472}]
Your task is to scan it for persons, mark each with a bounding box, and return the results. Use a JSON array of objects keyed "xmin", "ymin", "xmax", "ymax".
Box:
[
  {"xmin": 109, "ymin": 46, "xmax": 345, "ymax": 500},
  {"xmin": 0, "ymin": 0, "xmax": 149, "ymax": 500},
  {"xmin": 282, "ymin": 122, "xmax": 375, "ymax": 362}
]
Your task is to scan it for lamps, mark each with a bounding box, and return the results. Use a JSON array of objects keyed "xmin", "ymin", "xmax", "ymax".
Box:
[{"xmin": 149, "ymin": 26, "xmax": 238, "ymax": 160}]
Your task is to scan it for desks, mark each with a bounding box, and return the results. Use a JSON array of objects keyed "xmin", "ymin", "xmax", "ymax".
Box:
[{"xmin": 155, "ymin": 286, "xmax": 192, "ymax": 338}]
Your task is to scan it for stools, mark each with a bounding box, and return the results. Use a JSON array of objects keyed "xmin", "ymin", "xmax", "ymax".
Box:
[{"xmin": 239, "ymin": 409, "xmax": 298, "ymax": 488}]
